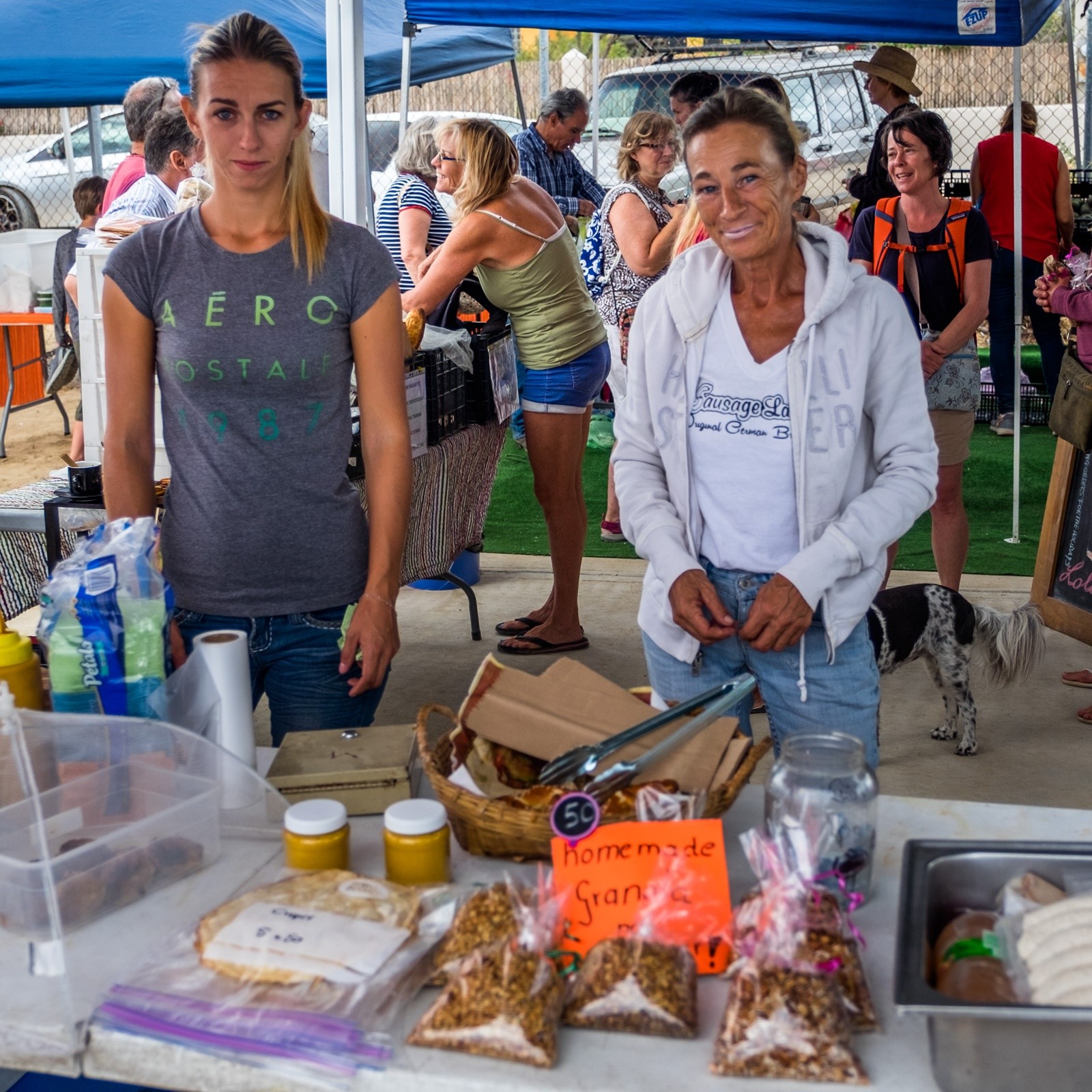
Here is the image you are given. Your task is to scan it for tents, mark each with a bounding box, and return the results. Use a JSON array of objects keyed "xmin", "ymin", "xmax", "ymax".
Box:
[
  {"xmin": 0, "ymin": 0, "xmax": 527, "ymax": 175},
  {"xmin": 396, "ymin": 0, "xmax": 1062, "ymax": 544}
]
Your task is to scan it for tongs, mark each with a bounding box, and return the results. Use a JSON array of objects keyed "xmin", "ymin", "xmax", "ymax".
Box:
[{"xmin": 537, "ymin": 671, "xmax": 756, "ymax": 806}]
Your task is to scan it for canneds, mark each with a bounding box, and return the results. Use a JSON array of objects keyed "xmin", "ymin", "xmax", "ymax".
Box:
[
  {"xmin": 283, "ymin": 798, "xmax": 351, "ymax": 871},
  {"xmin": 384, "ymin": 797, "xmax": 454, "ymax": 887}
]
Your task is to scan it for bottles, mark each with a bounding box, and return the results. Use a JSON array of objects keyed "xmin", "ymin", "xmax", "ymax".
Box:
[
  {"xmin": 0, "ymin": 608, "xmax": 51, "ymax": 820},
  {"xmin": 763, "ymin": 731, "xmax": 878, "ymax": 911}
]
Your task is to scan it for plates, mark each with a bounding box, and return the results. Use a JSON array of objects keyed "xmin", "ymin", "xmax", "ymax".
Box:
[{"xmin": 53, "ymin": 487, "xmax": 103, "ymax": 501}]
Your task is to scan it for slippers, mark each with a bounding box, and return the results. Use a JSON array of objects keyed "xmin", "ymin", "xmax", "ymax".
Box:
[
  {"xmin": 497, "ymin": 636, "xmax": 589, "ymax": 654},
  {"xmin": 495, "ymin": 618, "xmax": 584, "ymax": 636}
]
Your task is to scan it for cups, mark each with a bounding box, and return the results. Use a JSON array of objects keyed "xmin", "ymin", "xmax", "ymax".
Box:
[{"xmin": 67, "ymin": 461, "xmax": 102, "ymax": 496}]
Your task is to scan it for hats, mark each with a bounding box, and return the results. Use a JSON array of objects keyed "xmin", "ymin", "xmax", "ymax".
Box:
[{"xmin": 853, "ymin": 46, "xmax": 922, "ymax": 97}]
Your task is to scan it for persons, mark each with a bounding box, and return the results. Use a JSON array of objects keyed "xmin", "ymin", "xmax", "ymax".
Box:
[
  {"xmin": 52, "ymin": 75, "xmax": 212, "ymax": 468},
  {"xmin": 1032, "ymin": 229, "xmax": 1092, "ymax": 725},
  {"xmin": 848, "ymin": 111, "xmax": 993, "ymax": 591},
  {"xmin": 396, "ymin": 119, "xmax": 611, "ymax": 652},
  {"xmin": 101, "ymin": 13, "xmax": 410, "ymax": 745},
  {"xmin": 609, "ymin": 87, "xmax": 937, "ymax": 796},
  {"xmin": 973, "ymin": 100, "xmax": 1076, "ymax": 435},
  {"xmin": 840, "ymin": 46, "xmax": 922, "ymax": 224},
  {"xmin": 375, "ymin": 75, "xmax": 821, "ymax": 540}
]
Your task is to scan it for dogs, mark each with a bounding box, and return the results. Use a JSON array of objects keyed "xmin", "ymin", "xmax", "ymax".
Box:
[{"xmin": 750, "ymin": 583, "xmax": 1048, "ymax": 756}]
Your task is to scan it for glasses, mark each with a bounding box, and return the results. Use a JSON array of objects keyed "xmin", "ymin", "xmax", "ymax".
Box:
[
  {"xmin": 159, "ymin": 77, "xmax": 170, "ymax": 111},
  {"xmin": 438, "ymin": 150, "xmax": 466, "ymax": 161},
  {"xmin": 638, "ymin": 141, "xmax": 676, "ymax": 153}
]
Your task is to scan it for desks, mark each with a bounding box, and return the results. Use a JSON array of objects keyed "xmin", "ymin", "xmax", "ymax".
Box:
[
  {"xmin": 0, "ymin": 745, "xmax": 1092, "ymax": 1092},
  {"xmin": 0, "ymin": 412, "xmax": 513, "ymax": 640},
  {"xmin": 0, "ymin": 312, "xmax": 70, "ymax": 459}
]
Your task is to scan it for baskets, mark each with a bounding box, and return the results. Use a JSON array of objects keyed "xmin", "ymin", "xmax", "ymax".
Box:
[{"xmin": 419, "ymin": 701, "xmax": 777, "ymax": 859}]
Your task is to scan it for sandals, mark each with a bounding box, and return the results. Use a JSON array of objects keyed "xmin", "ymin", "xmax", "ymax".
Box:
[
  {"xmin": 1061, "ymin": 668, "xmax": 1092, "ymax": 688},
  {"xmin": 1077, "ymin": 716, "xmax": 1092, "ymax": 724}
]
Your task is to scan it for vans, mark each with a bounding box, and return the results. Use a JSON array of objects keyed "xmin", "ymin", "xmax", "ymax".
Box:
[{"xmin": 566, "ymin": 37, "xmax": 915, "ymax": 236}]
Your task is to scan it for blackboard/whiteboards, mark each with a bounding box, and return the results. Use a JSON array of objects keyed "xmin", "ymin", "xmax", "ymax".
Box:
[{"xmin": 1031, "ymin": 436, "xmax": 1091, "ymax": 647}]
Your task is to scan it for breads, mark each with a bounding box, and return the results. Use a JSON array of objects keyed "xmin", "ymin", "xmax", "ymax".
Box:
[
  {"xmin": 404, "ymin": 307, "xmax": 425, "ymax": 350},
  {"xmin": 494, "ymin": 744, "xmax": 680, "ymax": 816},
  {"xmin": 0, "ymin": 837, "xmax": 204, "ymax": 928}
]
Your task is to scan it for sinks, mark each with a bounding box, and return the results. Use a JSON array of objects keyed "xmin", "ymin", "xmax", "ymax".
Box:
[{"xmin": 891, "ymin": 835, "xmax": 1091, "ymax": 1021}]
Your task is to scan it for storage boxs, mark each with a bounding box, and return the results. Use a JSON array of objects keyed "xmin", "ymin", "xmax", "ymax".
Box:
[
  {"xmin": 344, "ymin": 324, "xmax": 511, "ymax": 482},
  {"xmin": 265, "ymin": 725, "xmax": 424, "ymax": 826},
  {"xmin": 975, "ymin": 380, "xmax": 1052, "ymax": 425},
  {"xmin": 78, "ymin": 246, "xmax": 173, "ymax": 480},
  {"xmin": 0, "ymin": 760, "xmax": 226, "ymax": 943},
  {"xmin": 0, "ymin": 228, "xmax": 73, "ymax": 291}
]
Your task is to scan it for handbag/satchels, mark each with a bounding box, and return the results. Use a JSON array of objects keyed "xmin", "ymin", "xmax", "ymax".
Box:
[
  {"xmin": 580, "ymin": 184, "xmax": 650, "ymax": 298},
  {"xmin": 1049, "ymin": 347, "xmax": 1092, "ymax": 452},
  {"xmin": 919, "ymin": 330, "xmax": 981, "ymax": 411}
]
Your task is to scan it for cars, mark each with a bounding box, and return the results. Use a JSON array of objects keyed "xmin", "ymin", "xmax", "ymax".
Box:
[
  {"xmin": 364, "ymin": 111, "xmax": 534, "ymax": 218},
  {"xmin": 0, "ymin": 100, "xmax": 326, "ymax": 232}
]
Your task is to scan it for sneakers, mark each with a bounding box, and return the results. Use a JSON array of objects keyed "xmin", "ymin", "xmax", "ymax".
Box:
[
  {"xmin": 990, "ymin": 412, "xmax": 1014, "ymax": 435},
  {"xmin": 601, "ymin": 513, "xmax": 626, "ymax": 543}
]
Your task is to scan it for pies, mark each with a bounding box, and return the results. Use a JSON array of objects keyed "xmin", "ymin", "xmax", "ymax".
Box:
[{"xmin": 193, "ymin": 868, "xmax": 421, "ymax": 985}]
